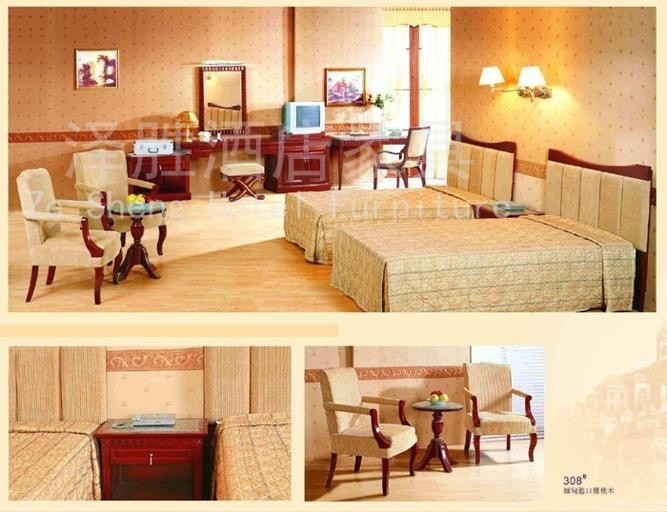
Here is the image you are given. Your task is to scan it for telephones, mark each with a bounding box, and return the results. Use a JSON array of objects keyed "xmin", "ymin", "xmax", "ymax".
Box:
[{"xmin": 494, "ymin": 200, "xmax": 525, "ymax": 211}]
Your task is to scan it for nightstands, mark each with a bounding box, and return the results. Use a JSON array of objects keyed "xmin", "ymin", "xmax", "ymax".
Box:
[
  {"xmin": 471, "ymin": 202, "xmax": 545, "ymax": 221},
  {"xmin": 92, "ymin": 418, "xmax": 209, "ymax": 501}
]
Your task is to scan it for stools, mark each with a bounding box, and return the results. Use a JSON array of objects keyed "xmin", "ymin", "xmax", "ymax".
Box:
[{"xmin": 218, "ymin": 162, "xmax": 267, "ymax": 202}]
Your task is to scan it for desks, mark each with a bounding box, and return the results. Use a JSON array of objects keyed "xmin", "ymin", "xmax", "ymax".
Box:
[
  {"xmin": 326, "ymin": 131, "xmax": 422, "ymax": 187},
  {"xmin": 128, "ymin": 132, "xmax": 331, "ymax": 201}
]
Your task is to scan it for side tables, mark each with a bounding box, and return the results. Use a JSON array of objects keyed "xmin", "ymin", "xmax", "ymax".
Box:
[
  {"xmin": 108, "ymin": 200, "xmax": 164, "ymax": 280},
  {"xmin": 411, "ymin": 399, "xmax": 463, "ymax": 470}
]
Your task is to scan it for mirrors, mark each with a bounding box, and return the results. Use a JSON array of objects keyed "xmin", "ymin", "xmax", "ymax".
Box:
[{"xmin": 199, "ymin": 66, "xmax": 250, "ymax": 134}]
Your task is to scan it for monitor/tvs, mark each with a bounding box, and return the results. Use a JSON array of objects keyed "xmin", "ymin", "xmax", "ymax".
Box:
[{"xmin": 282, "ymin": 102, "xmax": 325, "ymax": 135}]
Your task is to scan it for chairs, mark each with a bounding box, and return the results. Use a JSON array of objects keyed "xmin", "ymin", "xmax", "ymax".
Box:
[
  {"xmin": 372, "ymin": 125, "xmax": 431, "ymax": 189},
  {"xmin": 71, "ymin": 149, "xmax": 167, "ymax": 267},
  {"xmin": 462, "ymin": 360, "xmax": 540, "ymax": 466},
  {"xmin": 207, "ymin": 102, "xmax": 241, "ymax": 130},
  {"xmin": 320, "ymin": 365, "xmax": 418, "ymax": 498},
  {"xmin": 16, "ymin": 167, "xmax": 121, "ymax": 306}
]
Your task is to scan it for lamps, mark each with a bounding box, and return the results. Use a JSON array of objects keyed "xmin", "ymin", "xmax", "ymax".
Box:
[
  {"xmin": 175, "ymin": 111, "xmax": 199, "ymax": 141},
  {"xmin": 478, "ymin": 65, "xmax": 552, "ymax": 103}
]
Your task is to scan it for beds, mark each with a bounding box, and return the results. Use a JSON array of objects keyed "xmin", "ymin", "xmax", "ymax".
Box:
[
  {"xmin": 283, "ymin": 130, "xmax": 518, "ymax": 266},
  {"xmin": 201, "ymin": 347, "xmax": 291, "ymax": 501},
  {"xmin": 329, "ymin": 148, "xmax": 652, "ymax": 313},
  {"xmin": 9, "ymin": 348, "xmax": 106, "ymax": 501}
]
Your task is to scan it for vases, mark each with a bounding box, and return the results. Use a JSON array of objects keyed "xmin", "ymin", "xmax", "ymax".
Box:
[{"xmin": 378, "ymin": 110, "xmax": 388, "ymax": 137}]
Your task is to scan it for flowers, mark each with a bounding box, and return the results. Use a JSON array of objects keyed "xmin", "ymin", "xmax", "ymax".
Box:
[{"xmin": 367, "ymin": 92, "xmax": 396, "ymax": 110}]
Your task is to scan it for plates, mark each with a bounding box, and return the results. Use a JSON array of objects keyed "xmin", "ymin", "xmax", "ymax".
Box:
[{"xmin": 429, "ymin": 401, "xmax": 447, "ymax": 404}]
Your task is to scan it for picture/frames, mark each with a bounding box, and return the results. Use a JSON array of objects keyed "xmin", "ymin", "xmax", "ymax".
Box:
[
  {"xmin": 325, "ymin": 68, "xmax": 369, "ymax": 108},
  {"xmin": 74, "ymin": 49, "xmax": 119, "ymax": 90}
]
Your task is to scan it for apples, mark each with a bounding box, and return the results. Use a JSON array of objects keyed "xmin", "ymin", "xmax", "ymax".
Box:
[
  {"xmin": 431, "ymin": 393, "xmax": 448, "ymax": 400},
  {"xmin": 127, "ymin": 194, "xmax": 145, "ymax": 203}
]
